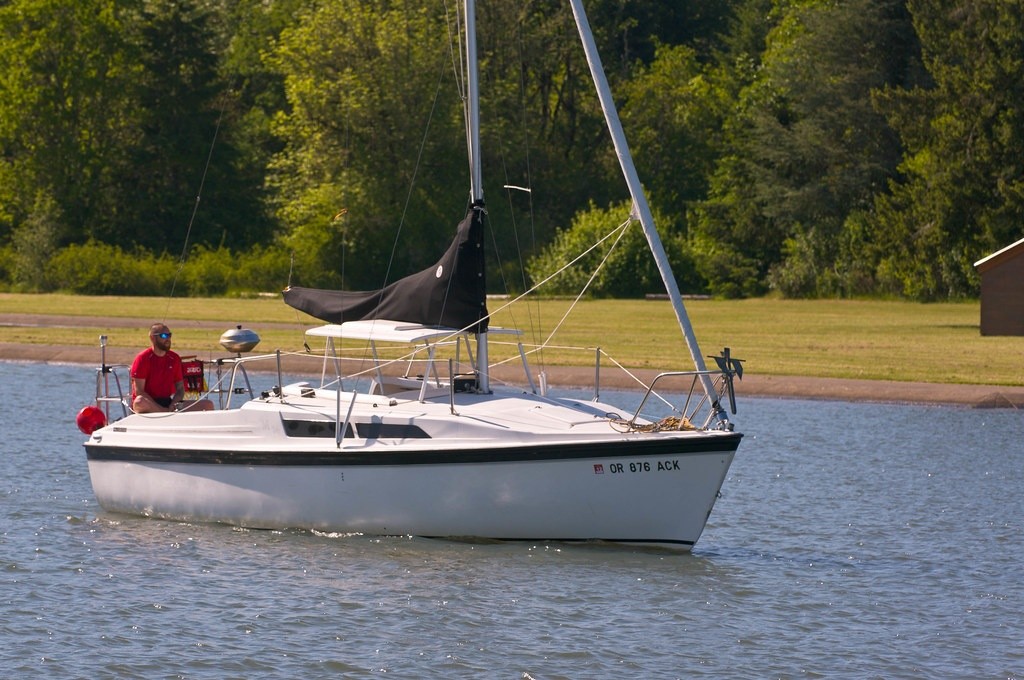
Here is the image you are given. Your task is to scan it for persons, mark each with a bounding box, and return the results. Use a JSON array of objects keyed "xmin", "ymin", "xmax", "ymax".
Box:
[{"xmin": 130, "ymin": 323, "xmax": 214, "ymax": 413}]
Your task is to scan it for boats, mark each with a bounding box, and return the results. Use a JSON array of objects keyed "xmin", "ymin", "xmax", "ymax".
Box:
[{"xmin": 81, "ymin": 320, "xmax": 744, "ymax": 552}]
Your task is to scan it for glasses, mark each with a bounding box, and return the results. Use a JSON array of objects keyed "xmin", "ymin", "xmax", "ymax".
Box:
[{"xmin": 153, "ymin": 333, "xmax": 173, "ymax": 339}]
